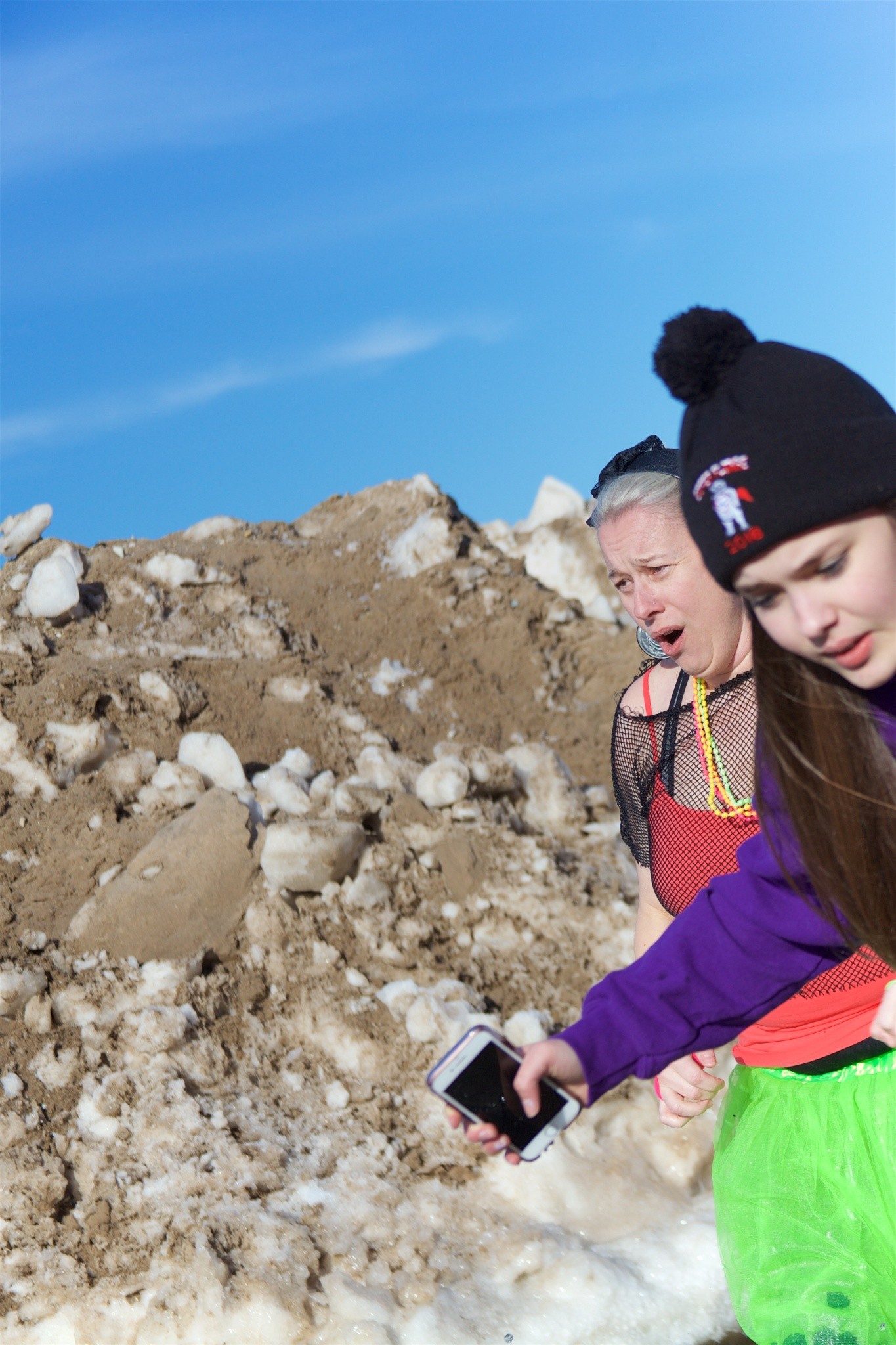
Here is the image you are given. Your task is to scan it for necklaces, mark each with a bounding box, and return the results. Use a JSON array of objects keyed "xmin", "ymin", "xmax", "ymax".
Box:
[{"xmin": 692, "ymin": 677, "xmax": 759, "ymax": 821}]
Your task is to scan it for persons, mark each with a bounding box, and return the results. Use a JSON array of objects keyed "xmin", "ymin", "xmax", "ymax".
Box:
[
  {"xmin": 449, "ymin": 307, "xmax": 896, "ymax": 1165},
  {"xmin": 585, "ymin": 434, "xmax": 896, "ymax": 1345}
]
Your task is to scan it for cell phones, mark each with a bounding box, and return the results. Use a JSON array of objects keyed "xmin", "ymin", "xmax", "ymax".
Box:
[{"xmin": 425, "ymin": 1024, "xmax": 582, "ymax": 1161}]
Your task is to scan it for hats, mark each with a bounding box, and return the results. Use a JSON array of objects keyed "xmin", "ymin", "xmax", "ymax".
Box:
[{"xmin": 651, "ymin": 305, "xmax": 896, "ymax": 592}]
